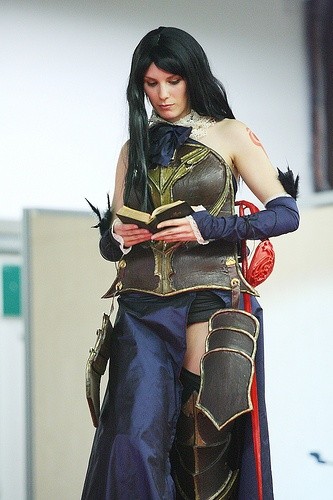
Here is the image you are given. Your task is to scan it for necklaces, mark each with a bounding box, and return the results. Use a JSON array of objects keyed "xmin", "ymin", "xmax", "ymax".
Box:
[{"xmin": 147, "ymin": 110, "xmax": 215, "ymax": 138}]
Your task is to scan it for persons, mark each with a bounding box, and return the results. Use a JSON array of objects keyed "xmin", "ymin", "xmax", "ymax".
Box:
[{"xmin": 78, "ymin": 26, "xmax": 299, "ymax": 499}]
